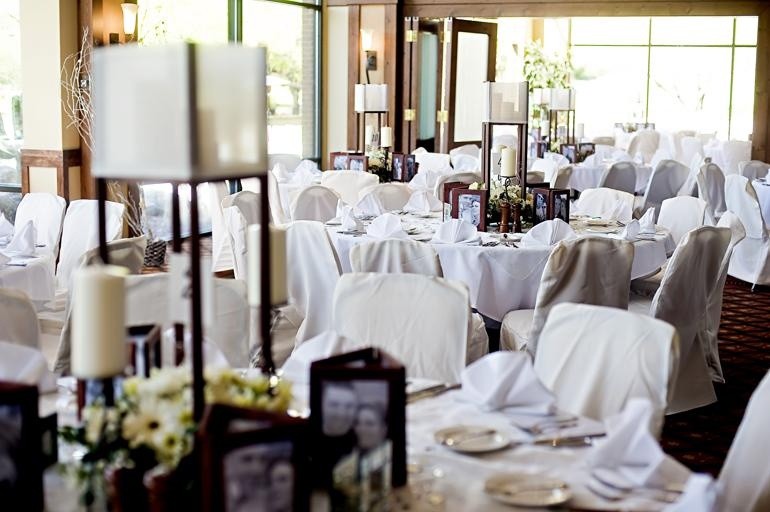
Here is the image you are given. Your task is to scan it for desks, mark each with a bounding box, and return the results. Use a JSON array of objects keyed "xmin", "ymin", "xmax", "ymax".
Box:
[
  {"xmin": 322, "ymin": 209, "xmax": 676, "ymax": 323},
  {"xmin": 1, "ymin": 240, "xmax": 58, "ymax": 314},
  {"xmin": 750, "ymin": 176, "xmax": 770, "ymax": 231},
  {"xmin": 38, "ymin": 369, "xmax": 720, "ymax": 512},
  {"xmin": 531, "ymin": 145, "xmax": 655, "ymax": 192}
]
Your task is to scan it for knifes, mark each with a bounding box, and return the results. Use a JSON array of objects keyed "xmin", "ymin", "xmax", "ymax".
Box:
[
  {"xmin": 4, "ymin": 263, "xmax": 27, "ymax": 266},
  {"xmin": 635, "ymin": 237, "xmax": 656, "ymax": 241},
  {"xmin": 406, "ymin": 380, "xmax": 465, "ymax": 404},
  {"xmin": 534, "ymin": 432, "xmax": 607, "ymax": 449}
]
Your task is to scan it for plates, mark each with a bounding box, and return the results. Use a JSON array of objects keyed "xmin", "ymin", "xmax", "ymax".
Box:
[
  {"xmin": 483, "ymin": 470, "xmax": 575, "ymax": 509},
  {"xmin": 342, "ymin": 207, "xmax": 618, "ymax": 247},
  {"xmin": 436, "ymin": 424, "xmax": 509, "ymax": 453}
]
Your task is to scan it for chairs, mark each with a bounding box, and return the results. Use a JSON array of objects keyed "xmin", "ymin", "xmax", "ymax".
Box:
[{"xmin": 0, "ymin": 127, "xmax": 770, "ymax": 512}]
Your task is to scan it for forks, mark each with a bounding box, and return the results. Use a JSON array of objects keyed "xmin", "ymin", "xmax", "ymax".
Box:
[
  {"xmin": 584, "ymin": 471, "xmax": 683, "ymax": 503},
  {"xmin": 511, "ymin": 415, "xmax": 580, "ymax": 435},
  {"xmin": 436, "ymin": 428, "xmax": 496, "ymax": 448},
  {"xmin": 615, "ymin": 219, "xmax": 626, "ymax": 227}
]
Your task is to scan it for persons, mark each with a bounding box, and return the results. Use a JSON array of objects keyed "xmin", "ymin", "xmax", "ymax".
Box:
[
  {"xmin": 535, "ymin": 193, "xmax": 569, "ymax": 224},
  {"xmin": 406, "ymin": 156, "xmax": 415, "ymax": 182},
  {"xmin": 458, "ymin": 194, "xmax": 480, "ymax": 228},
  {"xmin": 222, "ymin": 377, "xmax": 395, "ymax": 511},
  {"xmin": 562, "ymin": 145, "xmax": 594, "ymax": 163},
  {"xmin": 392, "ymin": 157, "xmax": 402, "ymax": 180}
]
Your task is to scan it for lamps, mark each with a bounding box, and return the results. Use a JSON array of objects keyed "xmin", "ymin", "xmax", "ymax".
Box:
[
  {"xmin": 354, "ymin": 84, "xmax": 388, "ymax": 154},
  {"xmin": 87, "ymin": 35, "xmax": 271, "ymax": 423},
  {"xmin": 480, "ymin": 80, "xmax": 529, "ymax": 196},
  {"xmin": 532, "ymin": 86, "xmax": 575, "ymax": 156}
]
